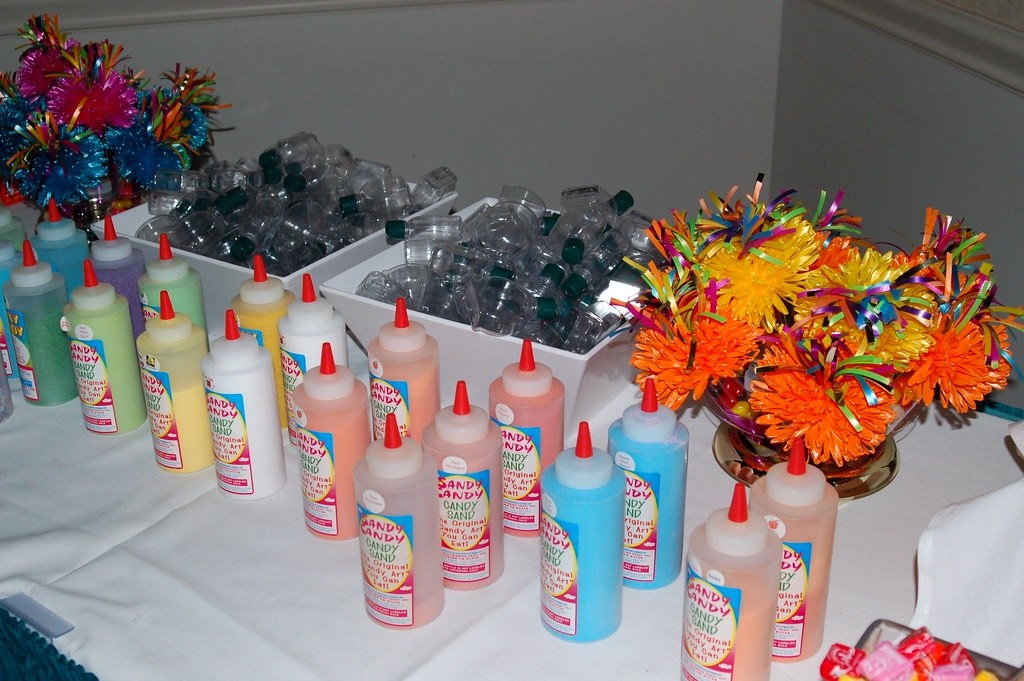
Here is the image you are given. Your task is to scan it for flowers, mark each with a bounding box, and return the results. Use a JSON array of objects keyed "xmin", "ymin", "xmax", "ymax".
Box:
[{"xmin": 612, "ymin": 171, "xmax": 1024, "ymax": 468}]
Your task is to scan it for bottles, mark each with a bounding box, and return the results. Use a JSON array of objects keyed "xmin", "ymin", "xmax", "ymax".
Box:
[
  {"xmin": 537, "ymin": 420, "xmax": 627, "ymax": 641},
  {"xmin": 681, "ymin": 484, "xmax": 781, "ymax": 680},
  {"xmin": 135, "ymin": 230, "xmax": 209, "ymax": 354},
  {"xmin": 4, "ymin": 241, "xmax": 78, "ymax": 406},
  {"xmin": 748, "ymin": 439, "xmax": 838, "ymax": 662},
  {"xmin": 230, "ymin": 252, "xmax": 298, "ymax": 428},
  {"xmin": 491, "ymin": 339, "xmax": 566, "ymax": 536},
  {"xmin": 33, "ymin": 197, "xmax": 89, "ymax": 301},
  {"xmin": 1, "ymin": 204, "xmax": 27, "ymax": 252},
  {"xmin": 0, "ymin": 240, "xmax": 24, "ymax": 379},
  {"xmin": 0, "ymin": 352, "xmax": 13, "ymax": 426},
  {"xmin": 84, "ymin": 213, "xmax": 148, "ymax": 336},
  {"xmin": 136, "ymin": 133, "xmax": 654, "ymax": 355},
  {"xmin": 278, "ymin": 274, "xmax": 350, "ymax": 453},
  {"xmin": 198, "ymin": 307, "xmax": 285, "ymax": 498},
  {"xmin": 62, "ymin": 260, "xmax": 147, "ymax": 436},
  {"xmin": 28, "ymin": 197, "xmax": 88, "ymax": 303},
  {"xmin": 134, "ymin": 289, "xmax": 216, "ymax": 472},
  {"xmin": 368, "ymin": 301, "xmax": 440, "ymax": 448},
  {"xmin": 353, "ymin": 412, "xmax": 443, "ymax": 629},
  {"xmin": 291, "ymin": 342, "xmax": 373, "ymax": 540},
  {"xmin": 419, "ymin": 379, "xmax": 505, "ymax": 590},
  {"xmin": 608, "ymin": 377, "xmax": 688, "ymax": 590}
]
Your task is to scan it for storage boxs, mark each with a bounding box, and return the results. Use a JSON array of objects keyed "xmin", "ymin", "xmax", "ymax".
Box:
[
  {"xmin": 321, "ymin": 197, "xmax": 644, "ymax": 440},
  {"xmin": 88, "ymin": 178, "xmax": 457, "ymax": 335}
]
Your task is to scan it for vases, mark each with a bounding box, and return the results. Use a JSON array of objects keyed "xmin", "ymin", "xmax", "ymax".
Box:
[{"xmin": 699, "ymin": 376, "xmax": 897, "ymax": 498}]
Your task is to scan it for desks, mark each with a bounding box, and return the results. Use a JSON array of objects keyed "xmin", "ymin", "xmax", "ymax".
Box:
[{"xmin": 0, "ymin": 408, "xmax": 1021, "ymax": 681}]
